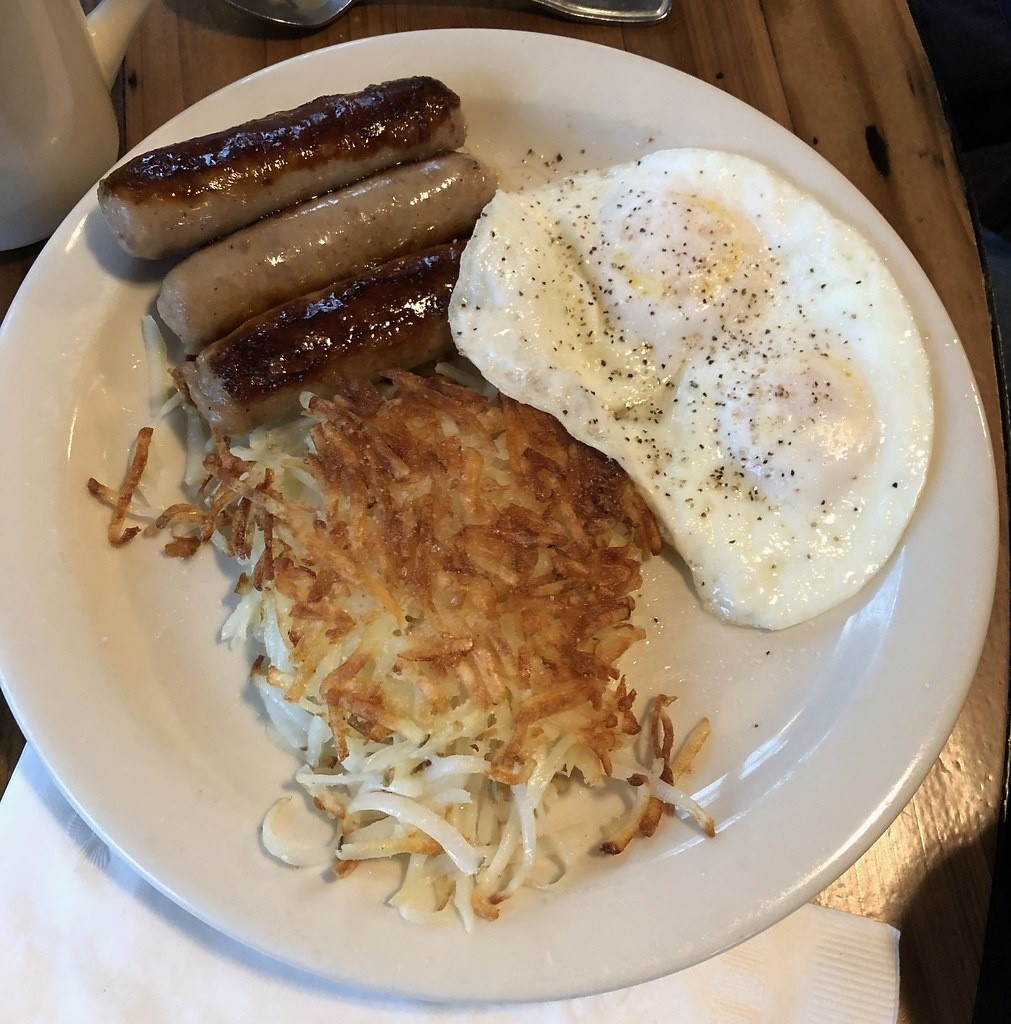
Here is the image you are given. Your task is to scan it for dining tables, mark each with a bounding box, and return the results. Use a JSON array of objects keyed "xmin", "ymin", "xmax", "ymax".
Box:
[{"xmin": 0, "ymin": 0, "xmax": 1011, "ymax": 919}]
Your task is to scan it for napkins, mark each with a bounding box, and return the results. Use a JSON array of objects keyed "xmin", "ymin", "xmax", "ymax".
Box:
[{"xmin": 0, "ymin": 740, "xmax": 901, "ymax": 1024}]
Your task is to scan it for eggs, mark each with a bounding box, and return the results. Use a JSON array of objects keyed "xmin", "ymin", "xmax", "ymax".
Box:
[{"xmin": 451, "ymin": 147, "xmax": 937, "ymax": 629}]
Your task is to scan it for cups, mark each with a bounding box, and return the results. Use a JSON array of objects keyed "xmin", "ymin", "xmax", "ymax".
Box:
[{"xmin": 0, "ymin": 0, "xmax": 153, "ymax": 256}]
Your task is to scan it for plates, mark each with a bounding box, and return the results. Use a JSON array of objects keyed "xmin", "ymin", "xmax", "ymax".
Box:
[{"xmin": 0, "ymin": 27, "xmax": 1000, "ymax": 1005}]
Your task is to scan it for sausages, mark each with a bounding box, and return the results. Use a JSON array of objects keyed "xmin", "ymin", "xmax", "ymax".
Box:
[{"xmin": 98, "ymin": 76, "xmax": 496, "ymax": 428}]
